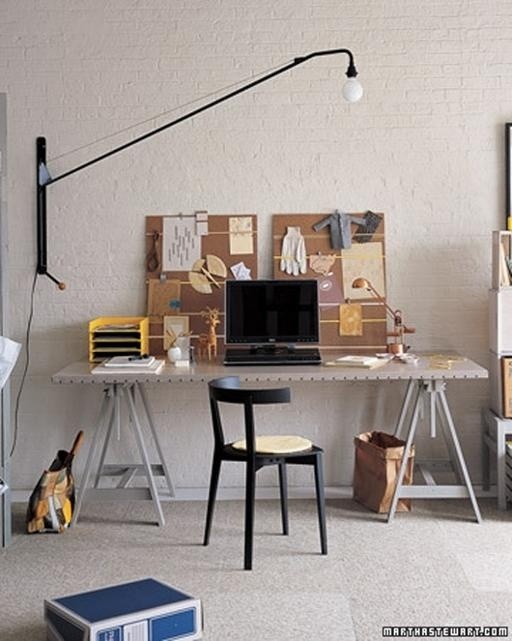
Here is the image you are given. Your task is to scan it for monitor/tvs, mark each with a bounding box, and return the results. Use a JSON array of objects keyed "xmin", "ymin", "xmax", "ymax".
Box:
[{"xmin": 225, "ymin": 279, "xmax": 320, "ymax": 346}]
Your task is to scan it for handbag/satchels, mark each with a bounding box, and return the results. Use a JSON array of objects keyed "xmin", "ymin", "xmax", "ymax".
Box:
[{"xmin": 25, "ymin": 449, "xmax": 76, "ymax": 534}]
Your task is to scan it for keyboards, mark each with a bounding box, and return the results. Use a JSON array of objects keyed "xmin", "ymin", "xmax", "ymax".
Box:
[{"xmin": 224, "ymin": 348, "xmax": 321, "ymax": 366}]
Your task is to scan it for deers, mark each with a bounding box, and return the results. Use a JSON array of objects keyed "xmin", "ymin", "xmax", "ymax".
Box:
[{"xmin": 195, "ymin": 305, "xmax": 221, "ymax": 361}]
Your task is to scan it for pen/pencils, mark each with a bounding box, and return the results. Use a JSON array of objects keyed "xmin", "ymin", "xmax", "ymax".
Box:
[{"xmin": 166, "ymin": 327, "xmax": 194, "ymax": 347}]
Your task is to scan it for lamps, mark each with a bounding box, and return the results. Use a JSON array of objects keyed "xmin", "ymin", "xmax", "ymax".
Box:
[
  {"xmin": 353, "ymin": 276, "xmax": 415, "ymax": 360},
  {"xmin": 27, "ymin": 46, "xmax": 361, "ymax": 296}
]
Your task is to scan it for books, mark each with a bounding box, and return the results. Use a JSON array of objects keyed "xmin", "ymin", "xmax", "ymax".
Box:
[
  {"xmin": 90, "ymin": 355, "xmax": 165, "ymax": 374},
  {"xmin": 324, "ymin": 355, "xmax": 389, "ymax": 370}
]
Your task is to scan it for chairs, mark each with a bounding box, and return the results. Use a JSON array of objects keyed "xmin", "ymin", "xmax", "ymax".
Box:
[{"xmin": 202, "ymin": 372, "xmax": 331, "ymax": 570}]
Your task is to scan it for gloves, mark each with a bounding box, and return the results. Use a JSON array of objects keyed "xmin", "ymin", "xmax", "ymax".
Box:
[{"xmin": 280, "ymin": 226, "xmax": 307, "ymax": 277}]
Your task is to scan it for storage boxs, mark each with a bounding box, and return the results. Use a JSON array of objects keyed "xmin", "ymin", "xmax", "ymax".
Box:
[{"xmin": 39, "ymin": 576, "xmax": 203, "ymax": 641}]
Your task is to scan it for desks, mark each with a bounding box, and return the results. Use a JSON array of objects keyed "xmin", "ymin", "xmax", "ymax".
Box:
[{"xmin": 52, "ymin": 349, "xmax": 488, "ymax": 530}]
